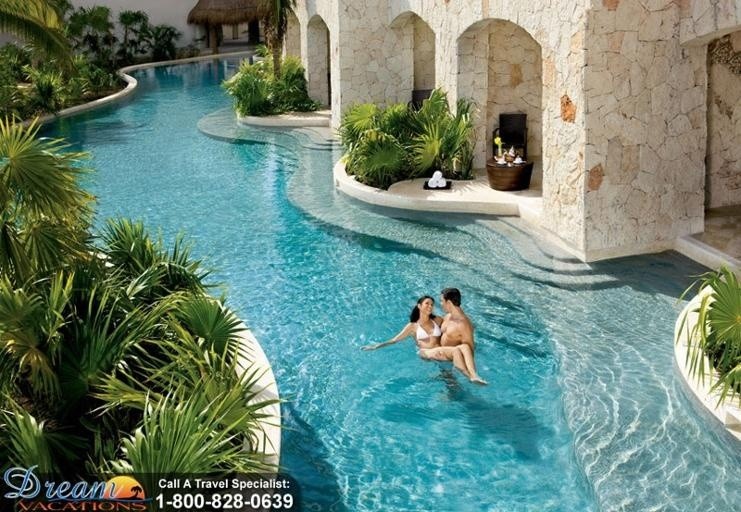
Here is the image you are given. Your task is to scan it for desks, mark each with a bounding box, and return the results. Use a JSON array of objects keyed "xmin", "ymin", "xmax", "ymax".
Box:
[{"xmin": 486, "ymin": 158, "xmax": 534, "ymax": 191}]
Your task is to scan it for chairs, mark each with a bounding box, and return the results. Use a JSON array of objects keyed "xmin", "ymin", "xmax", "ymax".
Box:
[{"xmin": 492, "ymin": 112, "xmax": 529, "ymax": 159}]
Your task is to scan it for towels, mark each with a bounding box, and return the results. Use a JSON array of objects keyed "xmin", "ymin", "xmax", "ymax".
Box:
[{"xmin": 428, "ymin": 170, "xmax": 447, "ymax": 187}]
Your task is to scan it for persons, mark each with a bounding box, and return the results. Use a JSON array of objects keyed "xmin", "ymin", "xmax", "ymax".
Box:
[{"xmin": 360, "ymin": 288, "xmax": 488, "ymax": 385}]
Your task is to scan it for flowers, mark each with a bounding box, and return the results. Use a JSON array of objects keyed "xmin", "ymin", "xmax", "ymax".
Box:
[{"xmin": 494, "ymin": 137, "xmax": 506, "ymax": 156}]
